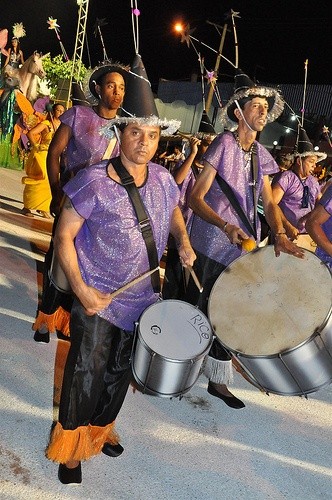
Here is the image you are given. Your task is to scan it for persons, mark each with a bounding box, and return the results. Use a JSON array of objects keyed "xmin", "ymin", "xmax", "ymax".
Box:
[
  {"xmin": 32, "ymin": 63, "xmax": 127, "ymax": 343},
  {"xmin": 159, "ymin": 144, "xmax": 185, "ymax": 177},
  {"xmin": 21, "ymin": 103, "xmax": 65, "ymax": 219},
  {"xmin": 185, "ymin": 68, "xmax": 305, "ymax": 410},
  {"xmin": 0, "ymin": 37, "xmax": 25, "ymax": 68},
  {"xmin": 162, "ymin": 110, "xmax": 218, "ymax": 299},
  {"xmin": 45, "ymin": 54, "xmax": 197, "ymax": 486},
  {"xmin": 257, "ymin": 122, "xmax": 332, "ymax": 275}
]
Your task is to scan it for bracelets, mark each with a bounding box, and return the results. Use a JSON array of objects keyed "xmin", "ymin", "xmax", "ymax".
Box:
[
  {"xmin": 275, "ymin": 233, "xmax": 287, "ymax": 241},
  {"xmin": 221, "ymin": 222, "xmax": 228, "ymax": 230}
]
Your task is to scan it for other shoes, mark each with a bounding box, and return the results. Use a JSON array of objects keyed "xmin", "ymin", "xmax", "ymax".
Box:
[
  {"xmin": 58, "ymin": 459, "xmax": 82, "ymax": 486},
  {"xmin": 36, "ymin": 210, "xmax": 50, "ymax": 219},
  {"xmin": 102, "ymin": 441, "xmax": 124, "ymax": 457},
  {"xmin": 207, "ymin": 381, "xmax": 246, "ymax": 409},
  {"xmin": 21, "ymin": 207, "xmax": 33, "ymax": 217},
  {"xmin": 34, "ymin": 328, "xmax": 49, "ymax": 344}
]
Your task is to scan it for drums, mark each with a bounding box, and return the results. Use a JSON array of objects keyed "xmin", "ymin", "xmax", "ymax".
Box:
[
  {"xmin": 293, "ymin": 234, "xmax": 318, "ymax": 253},
  {"xmin": 130, "ymin": 299, "xmax": 214, "ymax": 398},
  {"xmin": 207, "ymin": 244, "xmax": 332, "ymax": 396}
]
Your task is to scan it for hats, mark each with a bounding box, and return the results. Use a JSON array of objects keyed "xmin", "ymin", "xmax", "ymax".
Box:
[
  {"xmin": 98, "ymin": 54, "xmax": 181, "ymax": 141},
  {"xmin": 216, "ymin": 68, "xmax": 285, "ymax": 132},
  {"xmin": 286, "ymin": 124, "xmax": 327, "ymax": 163},
  {"xmin": 88, "ymin": 59, "xmax": 129, "ymax": 100}
]
